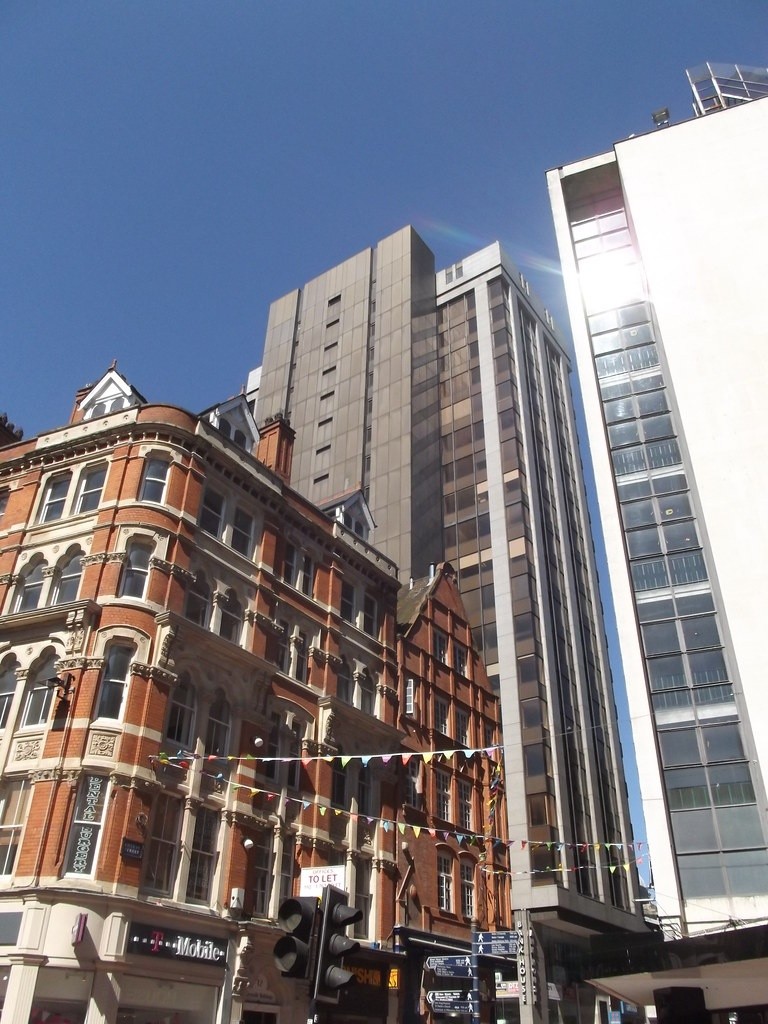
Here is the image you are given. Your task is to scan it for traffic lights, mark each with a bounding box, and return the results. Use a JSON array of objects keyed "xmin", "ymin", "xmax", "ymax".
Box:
[
  {"xmin": 312, "ymin": 886, "xmax": 364, "ymax": 1009},
  {"xmin": 273, "ymin": 895, "xmax": 317, "ymax": 983}
]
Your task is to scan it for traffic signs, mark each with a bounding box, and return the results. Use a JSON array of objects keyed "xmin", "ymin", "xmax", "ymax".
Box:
[{"xmin": 424, "ymin": 929, "xmax": 520, "ymax": 1024}]
